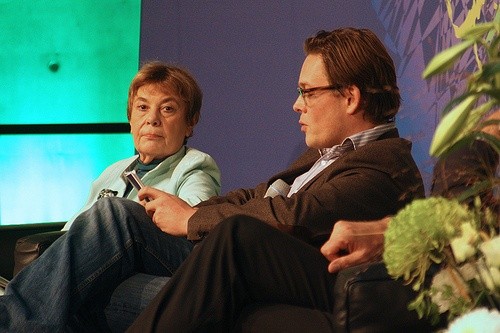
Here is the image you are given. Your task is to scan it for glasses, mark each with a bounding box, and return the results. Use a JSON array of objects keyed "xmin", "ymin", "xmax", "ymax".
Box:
[{"xmin": 296, "ymin": 84, "xmax": 344, "ymax": 97}]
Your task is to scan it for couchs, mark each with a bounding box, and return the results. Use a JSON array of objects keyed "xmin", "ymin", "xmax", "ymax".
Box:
[{"xmin": 12, "ymin": 230, "xmax": 436, "ymax": 333}]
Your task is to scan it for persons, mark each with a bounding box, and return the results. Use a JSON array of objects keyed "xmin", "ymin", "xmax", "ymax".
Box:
[
  {"xmin": 1, "ymin": 26, "xmax": 425, "ymax": 332},
  {"xmin": 60, "ymin": 61, "xmax": 221, "ymax": 231},
  {"xmin": 121, "ymin": 109, "xmax": 499, "ymax": 333}
]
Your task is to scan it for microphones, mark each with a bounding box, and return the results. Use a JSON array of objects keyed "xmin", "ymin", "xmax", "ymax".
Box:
[{"xmin": 263, "ymin": 178, "xmax": 292, "ymax": 199}]
